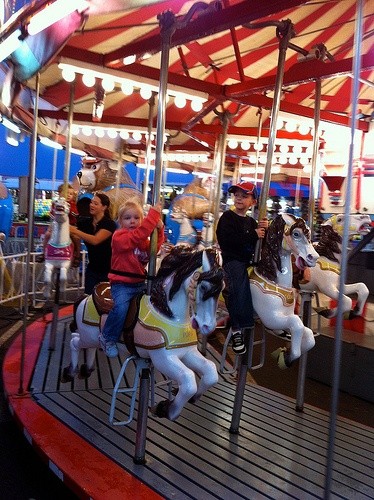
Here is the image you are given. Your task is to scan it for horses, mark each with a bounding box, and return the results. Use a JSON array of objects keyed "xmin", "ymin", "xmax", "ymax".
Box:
[
  {"xmin": 61, "ymin": 245, "xmax": 226, "ymax": 421},
  {"xmin": 216, "ymin": 213, "xmax": 320, "ymax": 368},
  {"xmin": 42, "ymin": 197, "xmax": 74, "ymax": 305},
  {"xmin": 298, "ymin": 214, "xmax": 371, "ymax": 320}
]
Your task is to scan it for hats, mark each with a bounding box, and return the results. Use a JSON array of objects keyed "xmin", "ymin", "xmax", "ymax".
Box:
[{"xmin": 228, "ymin": 180, "xmax": 259, "ymax": 199}]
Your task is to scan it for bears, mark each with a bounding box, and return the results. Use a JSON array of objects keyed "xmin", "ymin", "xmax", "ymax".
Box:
[
  {"xmin": 76, "ymin": 155, "xmax": 144, "ymax": 223},
  {"xmin": 165, "ymin": 177, "xmax": 223, "ymax": 246}
]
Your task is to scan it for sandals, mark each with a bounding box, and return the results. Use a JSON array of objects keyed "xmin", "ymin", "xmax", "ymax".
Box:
[
  {"xmin": 35, "ymin": 254, "xmax": 45, "ymax": 263},
  {"xmin": 71, "ymin": 255, "xmax": 82, "ymax": 268}
]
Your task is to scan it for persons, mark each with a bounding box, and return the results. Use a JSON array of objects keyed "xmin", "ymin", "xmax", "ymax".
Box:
[
  {"xmin": 36, "ymin": 185, "xmax": 81, "ymax": 267},
  {"xmin": 99, "ymin": 200, "xmax": 165, "ymax": 358},
  {"xmin": 215, "ymin": 183, "xmax": 270, "ymax": 355},
  {"xmin": 69, "ymin": 192, "xmax": 117, "ymax": 295}
]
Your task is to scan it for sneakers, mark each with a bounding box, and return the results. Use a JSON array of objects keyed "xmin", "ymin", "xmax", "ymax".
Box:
[
  {"xmin": 231, "ymin": 330, "xmax": 247, "ymax": 355},
  {"xmin": 264, "ymin": 326, "xmax": 292, "ymax": 342},
  {"xmin": 98, "ymin": 332, "xmax": 119, "ymax": 358}
]
